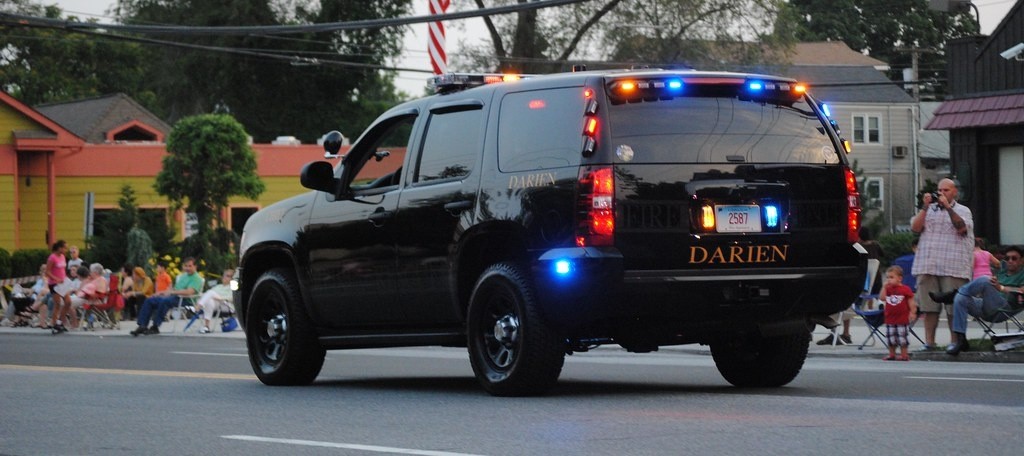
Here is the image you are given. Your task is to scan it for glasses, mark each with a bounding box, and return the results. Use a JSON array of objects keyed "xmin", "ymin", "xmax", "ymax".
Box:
[
  {"xmin": 1004, "ymin": 256, "xmax": 1021, "ymax": 261},
  {"xmin": 225, "ymin": 275, "xmax": 232, "ymax": 278}
]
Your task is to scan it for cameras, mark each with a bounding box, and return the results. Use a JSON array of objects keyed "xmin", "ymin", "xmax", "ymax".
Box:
[{"xmin": 931, "ymin": 192, "xmax": 939, "ymax": 203}]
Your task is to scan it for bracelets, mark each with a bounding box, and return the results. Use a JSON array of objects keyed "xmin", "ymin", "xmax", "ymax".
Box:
[
  {"xmin": 1000, "ymin": 285, "xmax": 1004, "ymax": 292},
  {"xmin": 922, "ymin": 205, "xmax": 929, "ymax": 211}
]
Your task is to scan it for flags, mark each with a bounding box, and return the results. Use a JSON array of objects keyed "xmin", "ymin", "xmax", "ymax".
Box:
[{"xmin": 427, "ymin": 0, "xmax": 451, "ymax": 75}]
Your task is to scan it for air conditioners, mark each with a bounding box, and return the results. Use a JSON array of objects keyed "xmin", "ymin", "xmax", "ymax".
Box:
[{"xmin": 892, "ymin": 146, "xmax": 905, "ymax": 157}]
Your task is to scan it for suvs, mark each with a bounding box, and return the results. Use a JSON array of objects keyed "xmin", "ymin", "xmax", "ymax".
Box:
[{"xmin": 230, "ymin": 62, "xmax": 870, "ymax": 398}]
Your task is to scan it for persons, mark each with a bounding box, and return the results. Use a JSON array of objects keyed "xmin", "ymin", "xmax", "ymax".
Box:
[
  {"xmin": 816, "ymin": 227, "xmax": 921, "ymax": 360},
  {"xmin": 910, "ymin": 178, "xmax": 975, "ymax": 351},
  {"xmin": 0, "ymin": 240, "xmax": 236, "ymax": 336},
  {"xmin": 928, "ymin": 237, "xmax": 1024, "ymax": 354}
]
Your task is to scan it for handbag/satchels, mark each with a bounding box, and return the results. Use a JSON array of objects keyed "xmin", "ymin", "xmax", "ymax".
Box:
[
  {"xmin": 110, "ymin": 293, "xmax": 125, "ymax": 312},
  {"xmin": 221, "ymin": 317, "xmax": 238, "ymax": 332}
]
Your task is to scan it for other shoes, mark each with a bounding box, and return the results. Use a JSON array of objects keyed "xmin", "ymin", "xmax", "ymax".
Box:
[
  {"xmin": 200, "ymin": 326, "xmax": 213, "ymax": 333},
  {"xmin": 119, "ymin": 313, "xmax": 169, "ymax": 321},
  {"xmin": 946, "ymin": 344, "xmax": 955, "ymax": 353},
  {"xmin": 185, "ymin": 306, "xmax": 196, "ymax": 315},
  {"xmin": 1, "ymin": 320, "xmax": 80, "ymax": 334},
  {"xmin": 896, "ymin": 355, "xmax": 908, "ymax": 361},
  {"xmin": 142, "ymin": 326, "xmax": 160, "ymax": 335},
  {"xmin": 952, "ymin": 340, "xmax": 970, "ymax": 356},
  {"xmin": 928, "ymin": 290, "xmax": 956, "ymax": 304},
  {"xmin": 816, "ymin": 334, "xmax": 852, "ymax": 345},
  {"xmin": 919, "ymin": 344, "xmax": 937, "ymax": 350},
  {"xmin": 130, "ymin": 327, "xmax": 149, "ymax": 334},
  {"xmin": 882, "ymin": 354, "xmax": 895, "ymax": 361}
]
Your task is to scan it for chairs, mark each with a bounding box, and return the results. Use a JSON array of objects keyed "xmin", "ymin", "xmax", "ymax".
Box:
[
  {"xmin": 77, "ymin": 275, "xmax": 119, "ymax": 331},
  {"xmin": 973, "ymin": 260, "xmax": 1024, "ymax": 348},
  {"xmin": 169, "ymin": 276, "xmax": 235, "ymax": 333},
  {"xmin": 814, "ymin": 254, "xmax": 925, "ymax": 350}
]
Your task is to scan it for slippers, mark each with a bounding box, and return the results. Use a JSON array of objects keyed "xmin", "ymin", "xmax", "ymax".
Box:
[
  {"xmin": 25, "ymin": 306, "xmax": 40, "ymax": 313},
  {"xmin": 20, "ymin": 312, "xmax": 33, "ymax": 320}
]
